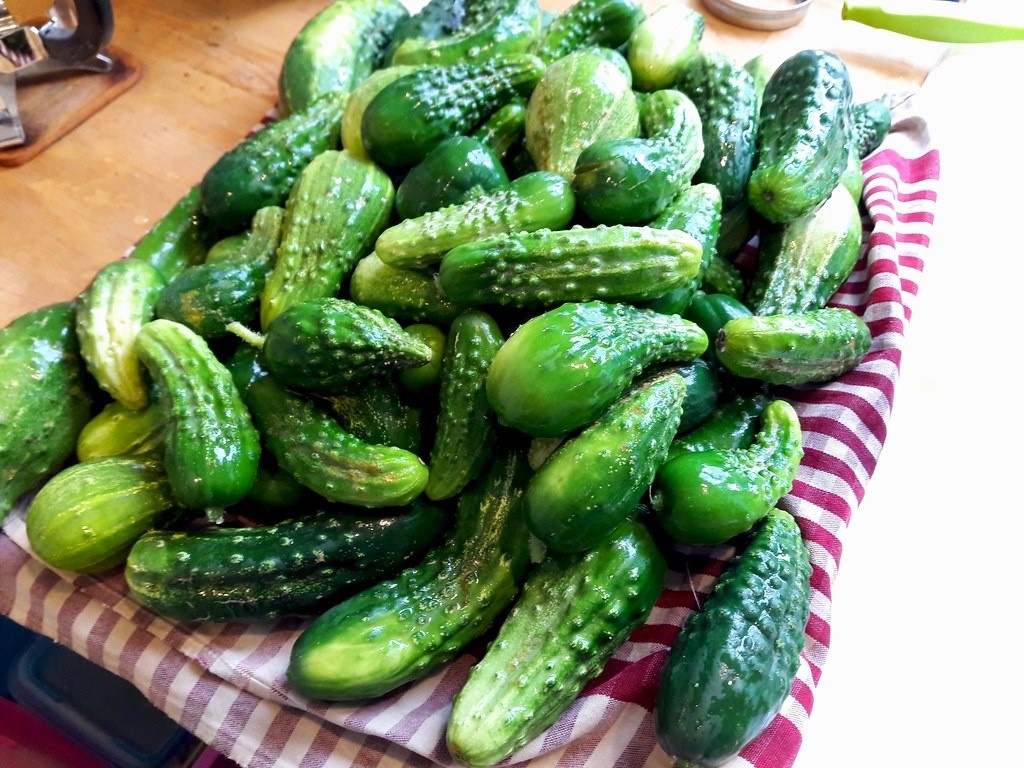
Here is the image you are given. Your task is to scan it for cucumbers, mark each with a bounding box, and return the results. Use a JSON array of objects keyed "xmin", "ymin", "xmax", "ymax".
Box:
[{"xmin": 0, "ymin": 0, "xmax": 892, "ymax": 765}]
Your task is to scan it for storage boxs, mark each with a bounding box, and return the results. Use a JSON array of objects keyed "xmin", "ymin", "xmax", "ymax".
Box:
[{"xmin": 7, "ymin": 632, "xmax": 199, "ymax": 768}]
